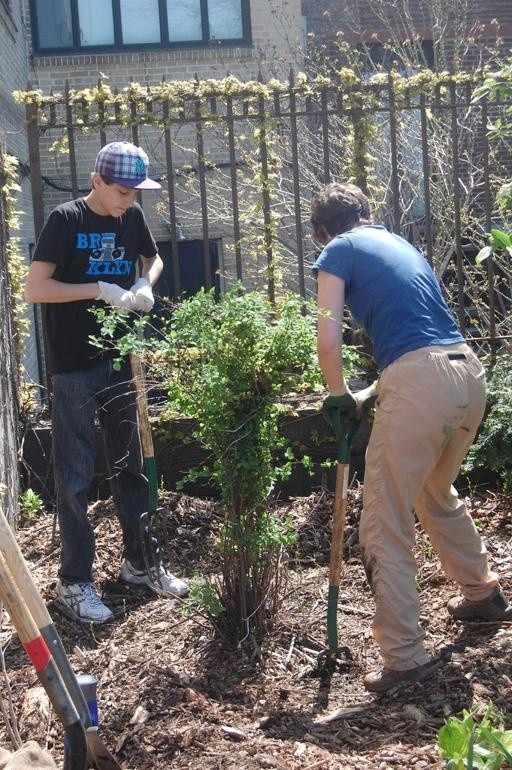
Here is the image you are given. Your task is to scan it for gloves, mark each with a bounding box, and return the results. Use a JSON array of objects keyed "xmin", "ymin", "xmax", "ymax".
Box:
[
  {"xmin": 95, "ymin": 281, "xmax": 136, "ymax": 310},
  {"xmin": 321, "ymin": 378, "xmax": 379, "ymax": 425},
  {"xmin": 131, "ymin": 277, "xmax": 155, "ymax": 313}
]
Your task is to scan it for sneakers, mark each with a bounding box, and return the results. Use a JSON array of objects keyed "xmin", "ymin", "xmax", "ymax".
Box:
[
  {"xmin": 120, "ymin": 557, "xmax": 190, "ymax": 599},
  {"xmin": 447, "ymin": 586, "xmax": 512, "ymax": 620},
  {"xmin": 53, "ymin": 578, "xmax": 116, "ymax": 626},
  {"xmin": 363, "ymin": 661, "xmax": 434, "ymax": 692}
]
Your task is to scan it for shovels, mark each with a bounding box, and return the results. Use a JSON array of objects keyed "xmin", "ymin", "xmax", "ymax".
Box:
[{"xmin": 1, "ymin": 558, "xmax": 121, "ymax": 770}]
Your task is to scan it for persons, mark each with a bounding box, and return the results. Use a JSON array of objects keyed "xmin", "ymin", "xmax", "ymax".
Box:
[
  {"xmin": 24, "ymin": 141, "xmax": 191, "ymax": 624},
  {"xmin": 309, "ymin": 182, "xmax": 510, "ymax": 694}
]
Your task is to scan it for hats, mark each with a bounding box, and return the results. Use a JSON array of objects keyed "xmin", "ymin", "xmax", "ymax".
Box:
[{"xmin": 95, "ymin": 141, "xmax": 162, "ymax": 190}]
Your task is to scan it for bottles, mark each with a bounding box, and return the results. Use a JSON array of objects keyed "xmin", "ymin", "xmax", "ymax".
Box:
[{"xmin": 63, "ymin": 677, "xmax": 98, "ymax": 760}]
